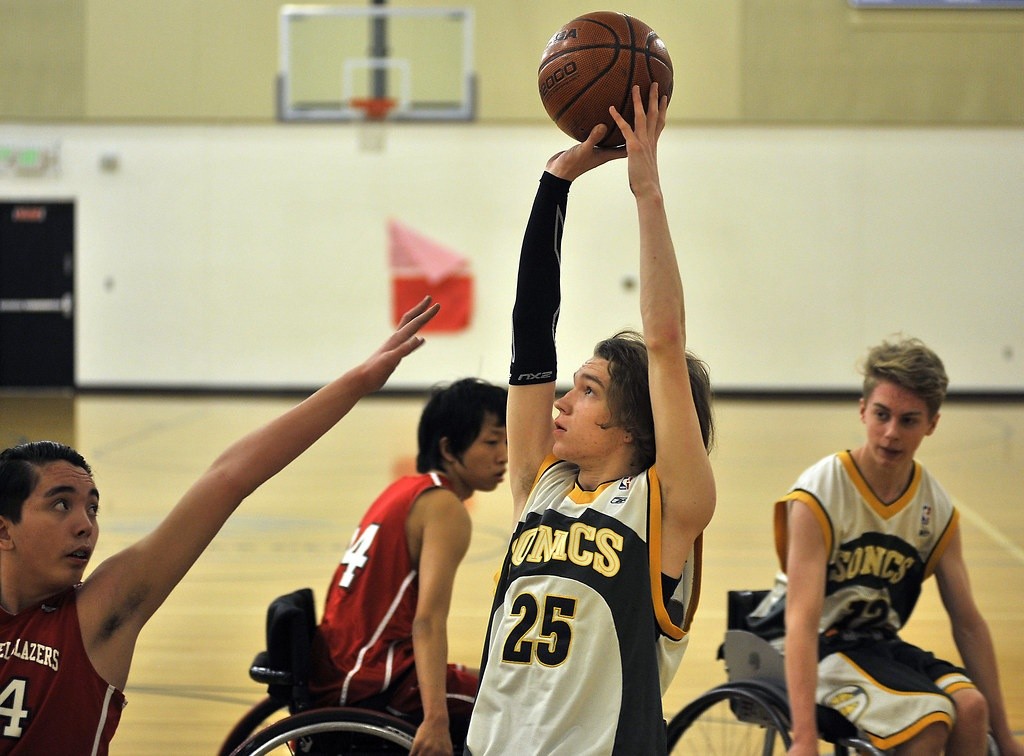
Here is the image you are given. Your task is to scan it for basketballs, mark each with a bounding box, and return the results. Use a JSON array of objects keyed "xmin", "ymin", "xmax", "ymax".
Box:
[{"xmin": 538, "ymin": 11, "xmax": 673, "ymax": 148}]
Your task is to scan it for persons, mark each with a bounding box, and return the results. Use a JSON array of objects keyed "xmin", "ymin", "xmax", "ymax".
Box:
[
  {"xmin": 772, "ymin": 337, "xmax": 1024, "ymax": 756},
  {"xmin": 0, "ymin": 295, "xmax": 439, "ymax": 756},
  {"xmin": 462, "ymin": 81, "xmax": 718, "ymax": 756},
  {"xmin": 318, "ymin": 377, "xmax": 508, "ymax": 756}
]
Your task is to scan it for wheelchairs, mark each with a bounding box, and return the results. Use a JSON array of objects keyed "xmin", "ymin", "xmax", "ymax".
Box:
[
  {"xmin": 215, "ymin": 588, "xmax": 462, "ymax": 755},
  {"xmin": 667, "ymin": 589, "xmax": 1000, "ymax": 756}
]
